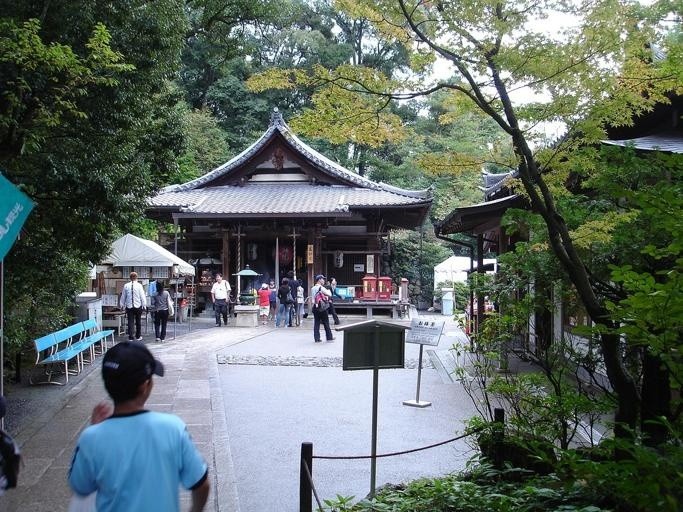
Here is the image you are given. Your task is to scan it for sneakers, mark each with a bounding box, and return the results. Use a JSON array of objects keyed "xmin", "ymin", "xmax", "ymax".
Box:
[
  {"xmin": 127, "ymin": 336, "xmax": 143, "ymax": 342},
  {"xmin": 156, "ymin": 337, "xmax": 165, "ymax": 343}
]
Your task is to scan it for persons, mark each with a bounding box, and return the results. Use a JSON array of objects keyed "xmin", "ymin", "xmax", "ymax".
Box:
[
  {"xmin": 249, "ymin": 286, "xmax": 257, "ymax": 306},
  {"xmin": 120, "ymin": 271, "xmax": 147, "ymax": 342},
  {"xmin": 210, "ymin": 272, "xmax": 232, "ymax": 327},
  {"xmin": 67, "ymin": 341, "xmax": 209, "ymax": 512},
  {"xmin": 148, "ymin": 278, "xmax": 174, "ymax": 342},
  {"xmin": 258, "ymin": 271, "xmax": 304, "ymax": 328},
  {"xmin": 311, "ymin": 275, "xmax": 344, "ymax": 342}
]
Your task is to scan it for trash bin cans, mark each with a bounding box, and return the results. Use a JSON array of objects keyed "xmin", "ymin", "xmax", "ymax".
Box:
[
  {"xmin": 178, "ymin": 299, "xmax": 188, "ymax": 322},
  {"xmin": 74, "ymin": 297, "xmax": 102, "ymax": 343},
  {"xmin": 442, "ymin": 288, "xmax": 453, "ymax": 315}
]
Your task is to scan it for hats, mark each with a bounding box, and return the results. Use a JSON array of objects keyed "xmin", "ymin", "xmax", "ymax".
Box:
[
  {"xmin": 102, "ymin": 341, "xmax": 164, "ymax": 391},
  {"xmin": 316, "ymin": 274, "xmax": 326, "ymax": 279}
]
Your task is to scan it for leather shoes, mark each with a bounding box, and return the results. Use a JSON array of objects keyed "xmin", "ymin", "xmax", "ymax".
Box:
[
  {"xmin": 327, "ymin": 337, "xmax": 335, "ymax": 340},
  {"xmin": 315, "ymin": 339, "xmax": 322, "ymax": 342}
]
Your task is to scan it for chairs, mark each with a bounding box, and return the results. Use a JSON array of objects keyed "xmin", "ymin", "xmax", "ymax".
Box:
[{"xmin": 31, "ymin": 317, "xmax": 115, "ymax": 386}]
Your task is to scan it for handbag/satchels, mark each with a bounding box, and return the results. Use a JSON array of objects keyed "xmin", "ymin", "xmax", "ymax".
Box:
[
  {"xmin": 286, "ymin": 287, "xmax": 296, "ymax": 303},
  {"xmin": 167, "ymin": 292, "xmax": 174, "ymax": 319}
]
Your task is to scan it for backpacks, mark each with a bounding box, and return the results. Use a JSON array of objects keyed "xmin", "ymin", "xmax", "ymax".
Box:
[{"xmin": 314, "ymin": 286, "xmax": 330, "ymax": 313}]
